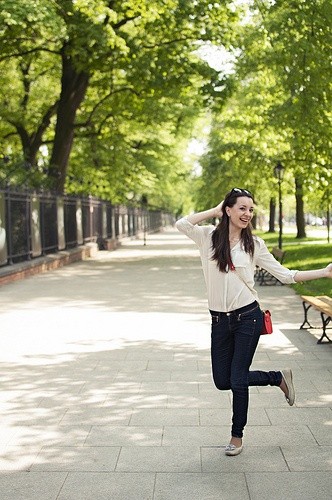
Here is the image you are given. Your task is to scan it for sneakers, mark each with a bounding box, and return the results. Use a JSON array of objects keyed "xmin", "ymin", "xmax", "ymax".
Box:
[
  {"xmin": 224, "ymin": 437, "xmax": 244, "ymax": 455},
  {"xmin": 279, "ymin": 368, "xmax": 295, "ymax": 406}
]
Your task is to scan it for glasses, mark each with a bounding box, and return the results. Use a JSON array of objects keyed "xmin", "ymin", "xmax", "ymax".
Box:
[{"xmin": 230, "ymin": 187, "xmax": 251, "ymax": 194}]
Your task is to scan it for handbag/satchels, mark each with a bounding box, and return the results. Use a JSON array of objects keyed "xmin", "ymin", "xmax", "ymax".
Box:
[{"xmin": 261, "ymin": 309, "xmax": 273, "ymax": 335}]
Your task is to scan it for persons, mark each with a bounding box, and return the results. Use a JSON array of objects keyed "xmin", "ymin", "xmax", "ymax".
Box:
[{"xmin": 176, "ymin": 189, "xmax": 332, "ymax": 456}]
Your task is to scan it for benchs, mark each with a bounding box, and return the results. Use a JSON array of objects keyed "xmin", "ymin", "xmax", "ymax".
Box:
[
  {"xmin": 300, "ymin": 295, "xmax": 332, "ymax": 344},
  {"xmin": 254, "ymin": 247, "xmax": 286, "ymax": 286}
]
